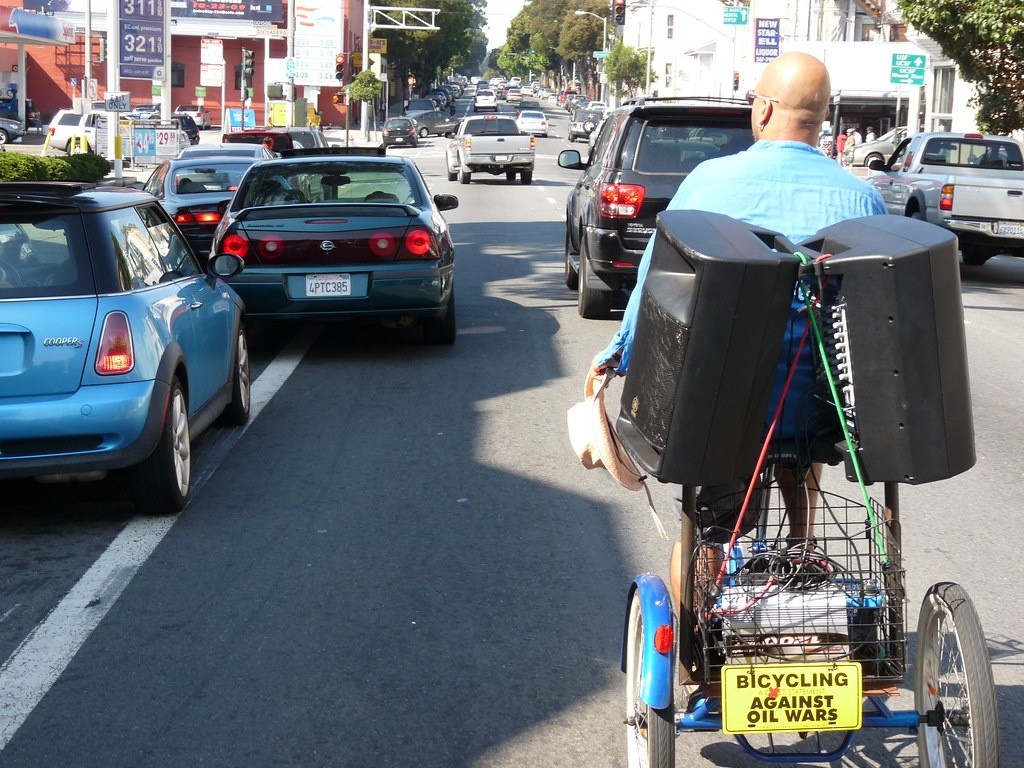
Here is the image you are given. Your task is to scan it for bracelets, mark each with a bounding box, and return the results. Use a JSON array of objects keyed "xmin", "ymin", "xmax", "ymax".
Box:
[{"xmin": 612, "ymin": 353, "xmax": 621, "ymax": 362}]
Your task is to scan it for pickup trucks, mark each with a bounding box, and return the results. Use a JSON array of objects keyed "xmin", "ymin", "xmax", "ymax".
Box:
[
  {"xmin": 445, "ymin": 113, "xmax": 536, "ymax": 185},
  {"xmin": 860, "ymin": 130, "xmax": 1024, "ymax": 265}
]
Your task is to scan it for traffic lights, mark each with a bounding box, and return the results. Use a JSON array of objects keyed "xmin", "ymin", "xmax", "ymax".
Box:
[
  {"xmin": 610, "ymin": 0, "xmax": 625, "ymax": 26},
  {"xmin": 243, "ymin": 50, "xmax": 255, "ymax": 76},
  {"xmin": 99, "ymin": 39, "xmax": 108, "ymax": 63},
  {"xmin": 335, "ymin": 53, "xmax": 346, "ymax": 81}
]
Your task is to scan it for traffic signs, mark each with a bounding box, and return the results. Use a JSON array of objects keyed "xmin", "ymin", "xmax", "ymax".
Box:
[
  {"xmin": 890, "ymin": 53, "xmax": 927, "ymax": 88},
  {"xmin": 722, "ymin": 5, "xmax": 748, "ymax": 27}
]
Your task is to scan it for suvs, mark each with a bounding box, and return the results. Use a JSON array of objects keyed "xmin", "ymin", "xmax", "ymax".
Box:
[
  {"xmin": 559, "ymin": 93, "xmax": 758, "ymax": 324},
  {"xmin": 567, "ymin": 107, "xmax": 602, "ymax": 143},
  {"xmin": 0, "ymin": 95, "xmax": 40, "ymax": 129}
]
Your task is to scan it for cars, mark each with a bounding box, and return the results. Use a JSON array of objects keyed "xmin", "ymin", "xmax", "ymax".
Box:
[
  {"xmin": 843, "ymin": 124, "xmax": 909, "ymax": 170},
  {"xmin": 406, "ymin": 74, "xmax": 615, "ymax": 118},
  {"xmin": 405, "ymin": 109, "xmax": 462, "ymax": 138},
  {"xmin": 206, "ymin": 145, "xmax": 458, "ymax": 347},
  {"xmin": 136, "ymin": 122, "xmax": 351, "ymax": 269},
  {"xmin": 380, "ymin": 116, "xmax": 420, "ymax": 149},
  {"xmin": 145, "ymin": 113, "xmax": 200, "ymax": 144},
  {"xmin": 516, "ymin": 108, "xmax": 550, "ymax": 138},
  {"xmin": 48, "ymin": 100, "xmax": 162, "ymax": 160},
  {"xmin": 0, "ymin": 116, "xmax": 26, "ymax": 144},
  {"xmin": 174, "ymin": 105, "xmax": 213, "ymax": 129},
  {"xmin": 0, "ymin": 178, "xmax": 250, "ymax": 516}
]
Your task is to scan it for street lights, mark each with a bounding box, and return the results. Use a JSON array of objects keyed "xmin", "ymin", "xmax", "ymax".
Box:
[{"xmin": 575, "ymin": 10, "xmax": 607, "ymax": 106}]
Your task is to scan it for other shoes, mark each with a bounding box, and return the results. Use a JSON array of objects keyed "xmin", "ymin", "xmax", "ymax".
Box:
[
  {"xmin": 785, "ymin": 535, "xmax": 835, "ymax": 577},
  {"xmin": 672, "ymin": 691, "xmax": 716, "ymax": 721}
]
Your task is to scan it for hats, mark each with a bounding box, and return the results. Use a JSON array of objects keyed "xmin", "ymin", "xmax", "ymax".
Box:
[
  {"xmin": 847, "ymin": 128, "xmax": 855, "ymax": 132},
  {"xmin": 565, "ymin": 367, "xmax": 649, "ymax": 494}
]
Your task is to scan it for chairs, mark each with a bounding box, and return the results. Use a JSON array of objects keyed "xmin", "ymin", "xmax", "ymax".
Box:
[
  {"xmin": 980, "ymin": 154, "xmax": 1002, "ymax": 167},
  {"xmin": 271, "ymin": 190, "xmax": 307, "ymax": 204},
  {"xmin": 183, "ymin": 183, "xmax": 208, "ymax": 193},
  {"xmin": 365, "ymin": 194, "xmax": 399, "ymax": 204}
]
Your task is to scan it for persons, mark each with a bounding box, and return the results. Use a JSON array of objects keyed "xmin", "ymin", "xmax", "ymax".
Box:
[
  {"xmin": 248, "ymin": 180, "xmax": 312, "ymax": 208},
  {"xmin": 262, "ymin": 137, "xmax": 278, "ymax": 158},
  {"xmin": 819, "ymin": 127, "xmax": 877, "ymax": 167},
  {"xmin": 37, "ymin": 112, "xmax": 41, "ymax": 132},
  {"xmin": 449, "ymin": 97, "xmax": 456, "ymax": 114},
  {"xmin": 891, "ymin": 143, "xmax": 913, "ymax": 171},
  {"xmin": 179, "ymin": 178, "xmax": 193, "ymax": 185},
  {"xmin": 594, "ymin": 52, "xmax": 888, "ymax": 686},
  {"xmin": 570, "ymin": 95, "xmax": 579, "ymax": 115}
]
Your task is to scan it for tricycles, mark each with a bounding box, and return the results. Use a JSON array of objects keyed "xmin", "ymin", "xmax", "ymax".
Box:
[{"xmin": 603, "ymin": 360, "xmax": 1003, "ymax": 768}]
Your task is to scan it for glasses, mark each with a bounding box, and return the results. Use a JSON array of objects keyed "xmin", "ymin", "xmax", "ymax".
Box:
[{"xmin": 746, "ymin": 90, "xmax": 780, "ymax": 105}]
